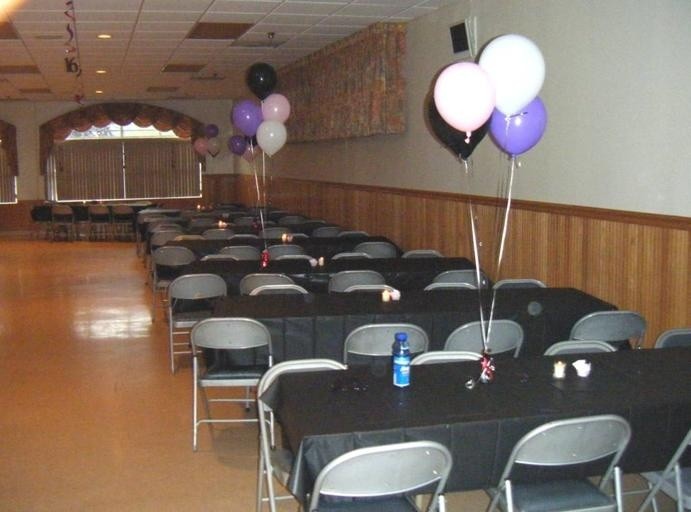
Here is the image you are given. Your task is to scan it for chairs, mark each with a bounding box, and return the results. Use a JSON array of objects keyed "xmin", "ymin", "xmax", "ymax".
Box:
[{"xmin": 26, "ymin": 199, "xmax": 137, "ymax": 244}]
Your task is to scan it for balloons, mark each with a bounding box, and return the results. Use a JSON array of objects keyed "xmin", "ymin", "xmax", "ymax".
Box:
[
  {"xmin": 427, "ymin": 32, "xmax": 550, "ymax": 353},
  {"xmin": 194, "ymin": 61, "xmax": 292, "ymax": 249}
]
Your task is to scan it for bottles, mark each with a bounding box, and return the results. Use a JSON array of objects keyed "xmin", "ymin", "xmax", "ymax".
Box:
[{"xmin": 391, "ymin": 332, "xmax": 410, "ymax": 386}]
